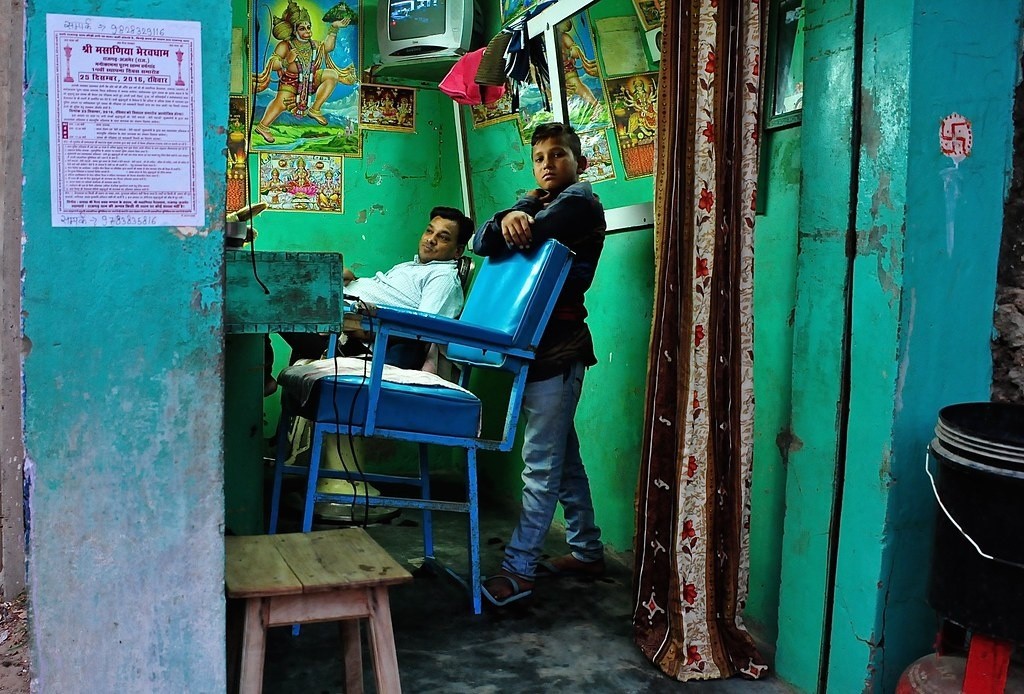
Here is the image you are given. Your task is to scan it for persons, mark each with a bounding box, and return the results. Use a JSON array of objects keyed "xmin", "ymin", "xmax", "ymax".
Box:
[
  {"xmin": 474, "ymin": 124, "xmax": 608, "ymax": 606},
  {"xmin": 255, "ymin": 206, "xmax": 473, "ymax": 465}
]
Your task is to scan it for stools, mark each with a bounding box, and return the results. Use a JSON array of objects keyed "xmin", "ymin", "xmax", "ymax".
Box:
[{"xmin": 215, "ymin": 523, "xmax": 414, "ymax": 694}]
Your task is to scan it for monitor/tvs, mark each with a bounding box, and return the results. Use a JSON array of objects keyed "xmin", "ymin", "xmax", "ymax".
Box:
[{"xmin": 376, "ymin": 0, "xmax": 486, "ymax": 66}]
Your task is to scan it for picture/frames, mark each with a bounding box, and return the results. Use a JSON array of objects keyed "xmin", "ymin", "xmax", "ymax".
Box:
[
  {"xmin": 254, "ymin": 150, "xmax": 347, "ymax": 214},
  {"xmin": 357, "ymin": 81, "xmax": 420, "ymax": 136},
  {"xmin": 225, "ymin": 95, "xmax": 249, "ymax": 215},
  {"xmin": 499, "ymin": 1, "xmax": 615, "ymax": 145},
  {"xmin": 630, "ymin": 0, "xmax": 666, "ymax": 30},
  {"xmin": 603, "ymin": 71, "xmax": 663, "ymax": 182},
  {"xmin": 240, "ymin": 1, "xmax": 367, "ymax": 160},
  {"xmin": 467, "ymin": 86, "xmax": 524, "ymax": 131},
  {"xmin": 570, "ymin": 133, "xmax": 617, "ymax": 187}
]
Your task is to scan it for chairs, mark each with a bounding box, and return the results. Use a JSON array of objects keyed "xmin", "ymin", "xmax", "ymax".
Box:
[
  {"xmin": 266, "ymin": 250, "xmax": 480, "ymax": 524},
  {"xmin": 258, "ymin": 232, "xmax": 575, "ymax": 640}
]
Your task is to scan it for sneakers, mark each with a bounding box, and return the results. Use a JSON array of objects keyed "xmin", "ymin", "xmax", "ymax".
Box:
[
  {"xmin": 481, "ymin": 568, "xmax": 534, "ymax": 605},
  {"xmin": 540, "ymin": 552, "xmax": 606, "ymax": 574}
]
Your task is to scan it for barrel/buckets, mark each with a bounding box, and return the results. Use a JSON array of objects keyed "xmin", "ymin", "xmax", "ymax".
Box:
[{"xmin": 924, "ymin": 402, "xmax": 1024, "ymax": 640}]
